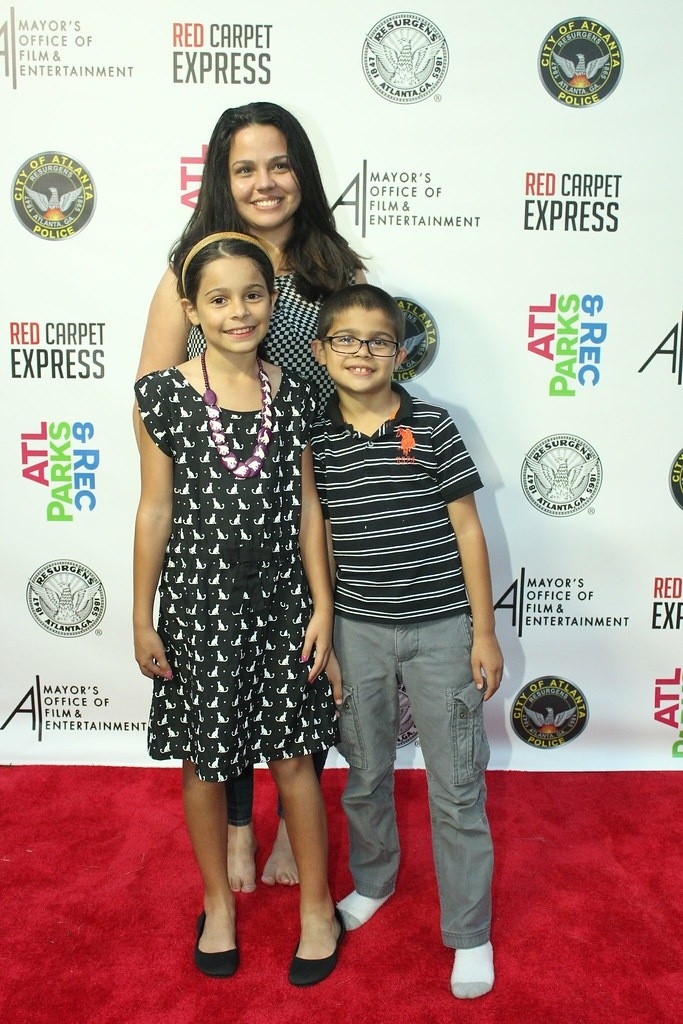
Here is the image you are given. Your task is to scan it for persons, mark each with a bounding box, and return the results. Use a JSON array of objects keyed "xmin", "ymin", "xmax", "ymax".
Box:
[
  {"xmin": 310, "ymin": 283, "xmax": 504, "ymax": 999},
  {"xmin": 132, "ymin": 101, "xmax": 367, "ymax": 894},
  {"xmin": 132, "ymin": 231, "xmax": 346, "ymax": 986}
]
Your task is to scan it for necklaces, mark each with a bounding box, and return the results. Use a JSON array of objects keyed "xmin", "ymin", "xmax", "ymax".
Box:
[{"xmin": 201, "ymin": 351, "xmax": 275, "ymax": 477}]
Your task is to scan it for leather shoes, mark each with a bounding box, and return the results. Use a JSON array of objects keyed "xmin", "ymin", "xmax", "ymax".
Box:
[
  {"xmin": 194, "ymin": 910, "xmax": 238, "ymax": 977},
  {"xmin": 287, "ymin": 907, "xmax": 346, "ymax": 987}
]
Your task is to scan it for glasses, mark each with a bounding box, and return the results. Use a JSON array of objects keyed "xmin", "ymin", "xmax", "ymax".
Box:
[{"xmin": 324, "ymin": 335, "xmax": 400, "ymax": 357}]
